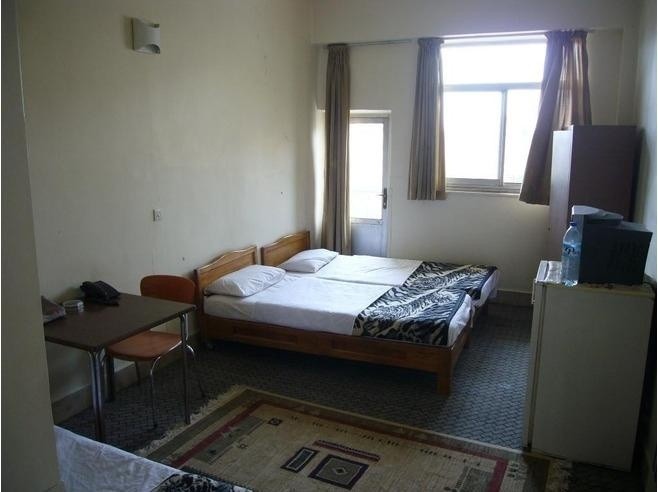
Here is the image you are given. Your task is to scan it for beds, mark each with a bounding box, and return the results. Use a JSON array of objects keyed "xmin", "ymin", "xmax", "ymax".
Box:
[
  {"xmin": 193, "ymin": 245, "xmax": 474, "ymax": 394},
  {"xmin": 260, "ymin": 230, "xmax": 501, "ymax": 329},
  {"xmin": 53, "ymin": 425, "xmax": 256, "ymax": 492}
]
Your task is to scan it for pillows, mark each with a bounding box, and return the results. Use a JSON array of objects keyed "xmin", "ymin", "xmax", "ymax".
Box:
[
  {"xmin": 207, "ymin": 264, "xmax": 286, "ymax": 297},
  {"xmin": 276, "ymin": 248, "xmax": 339, "ymax": 272}
]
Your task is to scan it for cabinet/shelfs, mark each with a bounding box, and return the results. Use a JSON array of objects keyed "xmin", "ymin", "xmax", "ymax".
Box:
[
  {"xmin": 546, "ymin": 124, "xmax": 637, "ymax": 261},
  {"xmin": 521, "ymin": 259, "xmax": 656, "ymax": 469}
]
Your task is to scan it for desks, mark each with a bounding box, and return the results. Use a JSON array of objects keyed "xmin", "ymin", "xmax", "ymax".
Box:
[{"xmin": 44, "ymin": 292, "xmax": 197, "ymax": 443}]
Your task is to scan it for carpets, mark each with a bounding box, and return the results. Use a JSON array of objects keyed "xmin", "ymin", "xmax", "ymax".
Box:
[{"xmin": 133, "ymin": 383, "xmax": 572, "ymax": 492}]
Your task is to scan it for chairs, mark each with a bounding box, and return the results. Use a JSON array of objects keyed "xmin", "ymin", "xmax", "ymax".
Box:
[{"xmin": 104, "ymin": 275, "xmax": 206, "ymax": 431}]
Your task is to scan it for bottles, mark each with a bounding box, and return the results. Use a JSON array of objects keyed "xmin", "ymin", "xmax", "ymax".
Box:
[{"xmin": 559, "ymin": 221, "xmax": 583, "ymax": 289}]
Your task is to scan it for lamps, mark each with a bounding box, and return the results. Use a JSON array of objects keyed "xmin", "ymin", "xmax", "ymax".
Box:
[{"xmin": 132, "ymin": 16, "xmax": 161, "ymax": 55}]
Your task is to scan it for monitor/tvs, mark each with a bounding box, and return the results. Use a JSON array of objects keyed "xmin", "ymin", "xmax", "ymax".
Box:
[{"xmin": 571, "ymin": 205, "xmax": 653, "ymax": 286}]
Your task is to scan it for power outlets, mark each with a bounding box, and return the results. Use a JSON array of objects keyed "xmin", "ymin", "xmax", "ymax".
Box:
[{"xmin": 153, "ymin": 208, "xmax": 164, "ymax": 222}]
